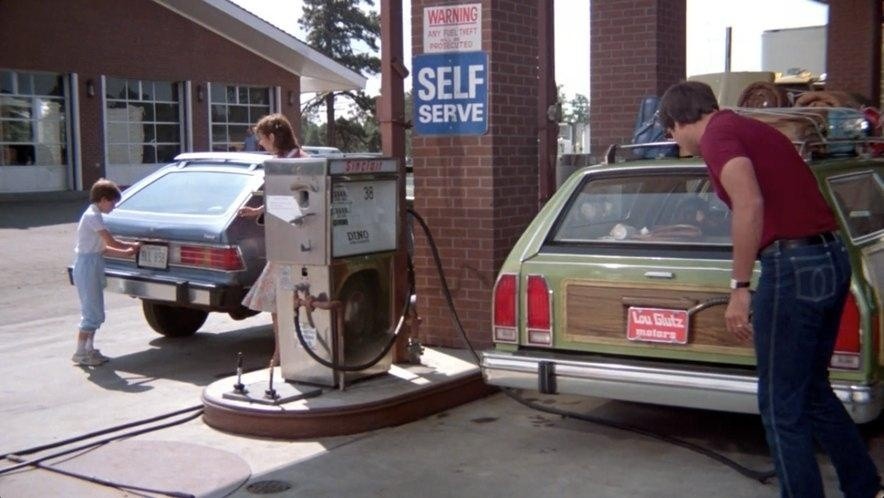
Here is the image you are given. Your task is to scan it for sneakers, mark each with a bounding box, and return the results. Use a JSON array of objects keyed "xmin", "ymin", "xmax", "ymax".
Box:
[{"xmin": 73, "ymin": 350, "xmax": 108, "ymax": 366}]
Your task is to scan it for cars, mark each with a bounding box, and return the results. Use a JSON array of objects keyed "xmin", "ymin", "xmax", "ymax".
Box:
[
  {"xmin": 58, "ymin": 139, "xmax": 416, "ymax": 345},
  {"xmin": 474, "ymin": 72, "xmax": 882, "ymax": 436}
]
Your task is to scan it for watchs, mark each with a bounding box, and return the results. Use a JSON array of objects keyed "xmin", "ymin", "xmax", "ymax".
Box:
[{"xmin": 729, "ymin": 278, "xmax": 752, "ymax": 291}]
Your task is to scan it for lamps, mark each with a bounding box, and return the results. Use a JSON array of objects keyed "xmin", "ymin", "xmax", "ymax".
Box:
[
  {"xmin": 87, "ymin": 79, "xmax": 94, "ymax": 96},
  {"xmin": 198, "ymin": 84, "xmax": 203, "ymax": 101},
  {"xmin": 288, "ymin": 90, "xmax": 294, "ymax": 104}
]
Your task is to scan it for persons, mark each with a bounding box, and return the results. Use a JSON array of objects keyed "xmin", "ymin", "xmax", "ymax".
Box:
[
  {"xmin": 655, "ymin": 79, "xmax": 884, "ymax": 498},
  {"xmin": 69, "ymin": 178, "xmax": 142, "ymax": 366},
  {"xmin": 243, "ymin": 127, "xmax": 257, "ymax": 150},
  {"xmin": 236, "ymin": 114, "xmax": 310, "ymax": 363}
]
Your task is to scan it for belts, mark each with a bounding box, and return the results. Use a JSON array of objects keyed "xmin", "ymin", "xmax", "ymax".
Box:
[{"xmin": 762, "ymin": 232, "xmax": 834, "ymax": 251}]
